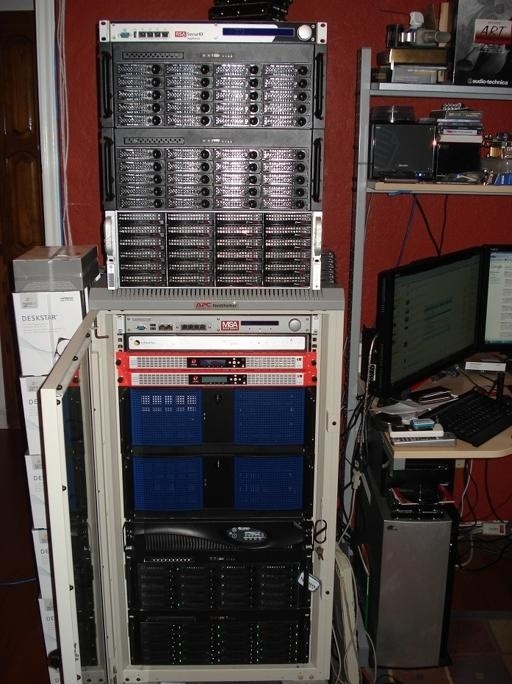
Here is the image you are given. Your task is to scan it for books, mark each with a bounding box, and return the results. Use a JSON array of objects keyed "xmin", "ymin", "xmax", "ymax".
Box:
[
  {"xmin": 392, "ymin": 484, "xmax": 458, "ymax": 506},
  {"xmin": 464, "ymin": 352, "xmax": 507, "ymax": 372},
  {"xmin": 419, "ymin": 109, "xmax": 485, "ymax": 144},
  {"xmin": 388, "ymin": 423, "xmax": 445, "ymax": 438}
]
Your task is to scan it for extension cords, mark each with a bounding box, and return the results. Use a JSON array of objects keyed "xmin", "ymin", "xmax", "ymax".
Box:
[{"xmin": 482, "ymin": 522, "xmax": 512, "ymax": 537}]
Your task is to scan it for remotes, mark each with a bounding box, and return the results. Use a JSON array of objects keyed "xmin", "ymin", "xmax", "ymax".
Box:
[{"xmin": 390, "ymin": 432, "xmax": 456, "ymax": 447}]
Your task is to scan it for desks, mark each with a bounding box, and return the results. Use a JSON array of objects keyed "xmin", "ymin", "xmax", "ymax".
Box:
[{"xmin": 367, "ymin": 353, "xmax": 511, "ymax": 476}]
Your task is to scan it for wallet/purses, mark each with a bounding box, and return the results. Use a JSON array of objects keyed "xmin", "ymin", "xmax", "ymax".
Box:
[{"xmin": 409, "ymin": 386, "xmax": 454, "ymax": 405}]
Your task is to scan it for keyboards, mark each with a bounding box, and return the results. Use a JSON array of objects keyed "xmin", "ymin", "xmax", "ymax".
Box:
[{"xmin": 424, "ymin": 389, "xmax": 511, "ymax": 447}]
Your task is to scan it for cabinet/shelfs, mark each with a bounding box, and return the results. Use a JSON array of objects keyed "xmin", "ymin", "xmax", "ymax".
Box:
[{"xmin": 340, "ymin": 45, "xmax": 512, "ymax": 546}]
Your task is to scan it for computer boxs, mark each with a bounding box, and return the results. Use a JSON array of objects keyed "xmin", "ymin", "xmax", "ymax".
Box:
[{"xmin": 354, "ymin": 477, "xmax": 456, "ymax": 670}]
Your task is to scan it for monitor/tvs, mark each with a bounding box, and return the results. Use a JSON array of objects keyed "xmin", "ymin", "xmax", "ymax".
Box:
[
  {"xmin": 483, "ymin": 246, "xmax": 512, "ymax": 368},
  {"xmin": 375, "ymin": 248, "xmax": 489, "ymax": 398}
]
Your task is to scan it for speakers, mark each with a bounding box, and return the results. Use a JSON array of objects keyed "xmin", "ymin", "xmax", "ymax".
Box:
[{"xmin": 370, "ymin": 123, "xmax": 438, "ymax": 183}]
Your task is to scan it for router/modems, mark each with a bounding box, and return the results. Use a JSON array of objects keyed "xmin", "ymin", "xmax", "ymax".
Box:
[{"xmin": 464, "ymin": 354, "xmax": 506, "ymax": 373}]
